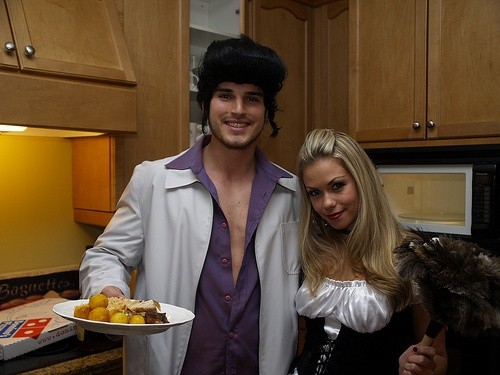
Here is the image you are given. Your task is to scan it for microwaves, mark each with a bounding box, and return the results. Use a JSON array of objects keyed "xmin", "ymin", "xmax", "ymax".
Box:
[{"xmin": 375, "ymin": 162, "xmax": 500, "ymax": 241}]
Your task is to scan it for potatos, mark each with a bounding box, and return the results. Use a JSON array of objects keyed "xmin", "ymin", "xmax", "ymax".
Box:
[{"xmin": 74, "ymin": 294, "xmax": 145, "ymax": 324}]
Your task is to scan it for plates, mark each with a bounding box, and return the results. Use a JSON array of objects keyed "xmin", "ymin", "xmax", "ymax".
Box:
[{"xmin": 52, "ymin": 299, "xmax": 195, "ymax": 335}]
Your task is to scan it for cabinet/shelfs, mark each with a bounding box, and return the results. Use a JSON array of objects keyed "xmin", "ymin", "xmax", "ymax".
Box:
[{"xmin": 0, "ymin": 1, "xmax": 500, "ymax": 230}]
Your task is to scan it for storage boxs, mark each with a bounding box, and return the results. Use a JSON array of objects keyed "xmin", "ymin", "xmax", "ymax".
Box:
[{"xmin": 0, "ymin": 297, "xmax": 85, "ymax": 362}]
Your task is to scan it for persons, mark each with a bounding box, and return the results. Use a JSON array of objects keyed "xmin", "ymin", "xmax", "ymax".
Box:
[
  {"xmin": 290, "ymin": 128, "xmax": 451, "ymax": 375},
  {"xmin": 78, "ymin": 35, "xmax": 301, "ymax": 375}
]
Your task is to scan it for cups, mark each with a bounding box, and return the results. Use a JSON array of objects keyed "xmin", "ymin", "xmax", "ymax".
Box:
[{"xmin": 189, "ymin": 54, "xmax": 199, "ymax": 88}]
